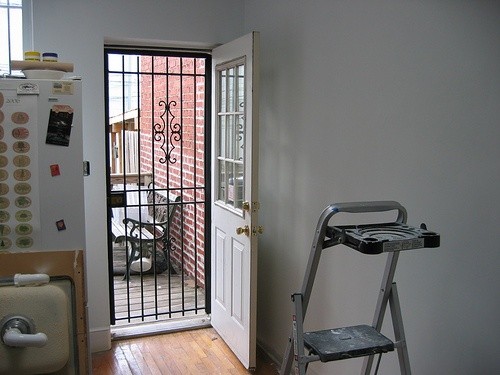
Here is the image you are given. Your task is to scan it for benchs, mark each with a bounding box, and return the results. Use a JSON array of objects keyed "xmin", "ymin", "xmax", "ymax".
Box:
[{"xmin": 110, "ymin": 182, "xmax": 182, "ymax": 281}]
